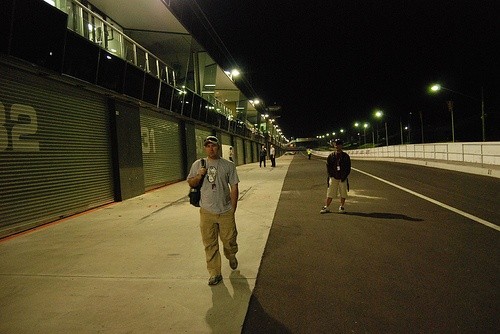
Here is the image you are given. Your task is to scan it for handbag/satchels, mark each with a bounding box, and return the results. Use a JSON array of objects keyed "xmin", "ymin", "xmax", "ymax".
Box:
[{"xmin": 187, "ymin": 158, "xmax": 208, "ymax": 207}]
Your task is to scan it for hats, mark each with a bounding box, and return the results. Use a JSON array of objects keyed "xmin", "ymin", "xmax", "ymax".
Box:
[{"xmin": 203, "ymin": 136, "xmax": 220, "ymax": 147}]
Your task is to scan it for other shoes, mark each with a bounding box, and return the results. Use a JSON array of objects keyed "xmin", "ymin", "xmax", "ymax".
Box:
[
  {"xmin": 339, "ymin": 205, "xmax": 345, "ymax": 213},
  {"xmin": 208, "ymin": 274, "xmax": 223, "ymax": 284},
  {"xmin": 229, "ymin": 257, "xmax": 238, "ymax": 270},
  {"xmin": 320, "ymin": 205, "xmax": 329, "ymax": 213}
]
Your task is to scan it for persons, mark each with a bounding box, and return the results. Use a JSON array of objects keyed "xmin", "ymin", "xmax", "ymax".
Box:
[
  {"xmin": 270, "ymin": 145, "xmax": 276, "ymax": 167},
  {"xmin": 319, "ymin": 139, "xmax": 352, "ymax": 213},
  {"xmin": 188, "ymin": 135, "xmax": 239, "ymax": 285},
  {"xmin": 259, "ymin": 145, "xmax": 267, "ymax": 167},
  {"xmin": 307, "ymin": 148, "xmax": 312, "ymax": 160}
]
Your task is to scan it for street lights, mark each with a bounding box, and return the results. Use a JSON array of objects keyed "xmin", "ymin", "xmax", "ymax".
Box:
[
  {"xmin": 376, "ymin": 110, "xmax": 388, "ymax": 145},
  {"xmin": 428, "ymin": 84, "xmax": 457, "ymax": 142},
  {"xmin": 362, "ymin": 121, "xmax": 370, "ymax": 148}
]
